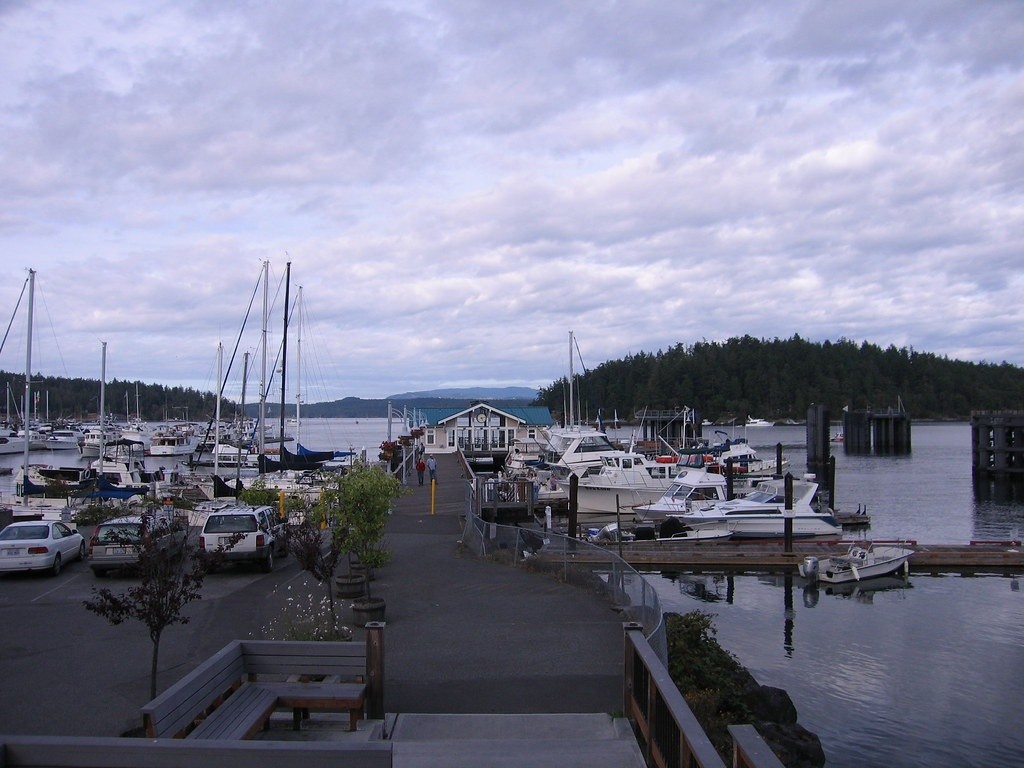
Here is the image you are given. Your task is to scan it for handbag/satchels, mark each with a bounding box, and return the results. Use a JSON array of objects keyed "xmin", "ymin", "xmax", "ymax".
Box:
[{"xmin": 419, "ymin": 451, "xmax": 422, "ymax": 455}]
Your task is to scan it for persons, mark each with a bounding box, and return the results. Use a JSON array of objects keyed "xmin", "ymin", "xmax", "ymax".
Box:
[
  {"xmin": 420, "ymin": 443, "xmax": 425, "ymax": 462},
  {"xmin": 426, "ymin": 454, "xmax": 438, "ymax": 485},
  {"xmin": 525, "ymin": 471, "xmax": 557, "ymax": 504},
  {"xmin": 416, "ymin": 458, "xmax": 425, "ymax": 486}
]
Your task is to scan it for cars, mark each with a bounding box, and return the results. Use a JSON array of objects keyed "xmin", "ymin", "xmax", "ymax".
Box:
[{"xmin": 0, "ymin": 520, "xmax": 87, "ymax": 579}]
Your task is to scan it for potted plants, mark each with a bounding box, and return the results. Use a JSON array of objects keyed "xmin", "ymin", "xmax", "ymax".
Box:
[{"xmin": 316, "ymin": 461, "xmax": 415, "ymax": 628}]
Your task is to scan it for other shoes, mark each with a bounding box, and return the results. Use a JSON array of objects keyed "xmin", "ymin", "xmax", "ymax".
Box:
[
  {"xmin": 422, "ymin": 483, "xmax": 423, "ymax": 486},
  {"xmin": 419, "ymin": 483, "xmax": 421, "ymax": 486}
]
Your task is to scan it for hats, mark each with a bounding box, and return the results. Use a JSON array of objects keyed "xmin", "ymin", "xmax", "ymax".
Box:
[{"xmin": 430, "ymin": 453, "xmax": 433, "ymax": 457}]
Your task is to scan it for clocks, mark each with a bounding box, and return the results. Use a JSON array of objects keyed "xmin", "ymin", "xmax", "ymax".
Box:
[{"xmin": 477, "ymin": 414, "xmax": 487, "ymax": 423}]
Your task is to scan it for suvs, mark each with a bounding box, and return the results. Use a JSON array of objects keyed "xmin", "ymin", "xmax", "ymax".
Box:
[
  {"xmin": 197, "ymin": 506, "xmax": 291, "ymax": 577},
  {"xmin": 87, "ymin": 517, "xmax": 189, "ymax": 580}
]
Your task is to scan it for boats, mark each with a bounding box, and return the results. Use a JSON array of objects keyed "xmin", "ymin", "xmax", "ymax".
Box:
[
  {"xmin": 796, "ymin": 542, "xmax": 916, "ymax": 585},
  {"xmin": 503, "ymin": 330, "xmax": 845, "ymax": 544},
  {"xmin": 0, "ymin": 258, "xmax": 359, "ymax": 506},
  {"xmin": 818, "ymin": 574, "xmax": 913, "ymax": 596}
]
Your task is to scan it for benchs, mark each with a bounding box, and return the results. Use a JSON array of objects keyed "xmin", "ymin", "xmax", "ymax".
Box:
[{"xmin": 138, "ymin": 639, "xmax": 368, "ymax": 741}]
